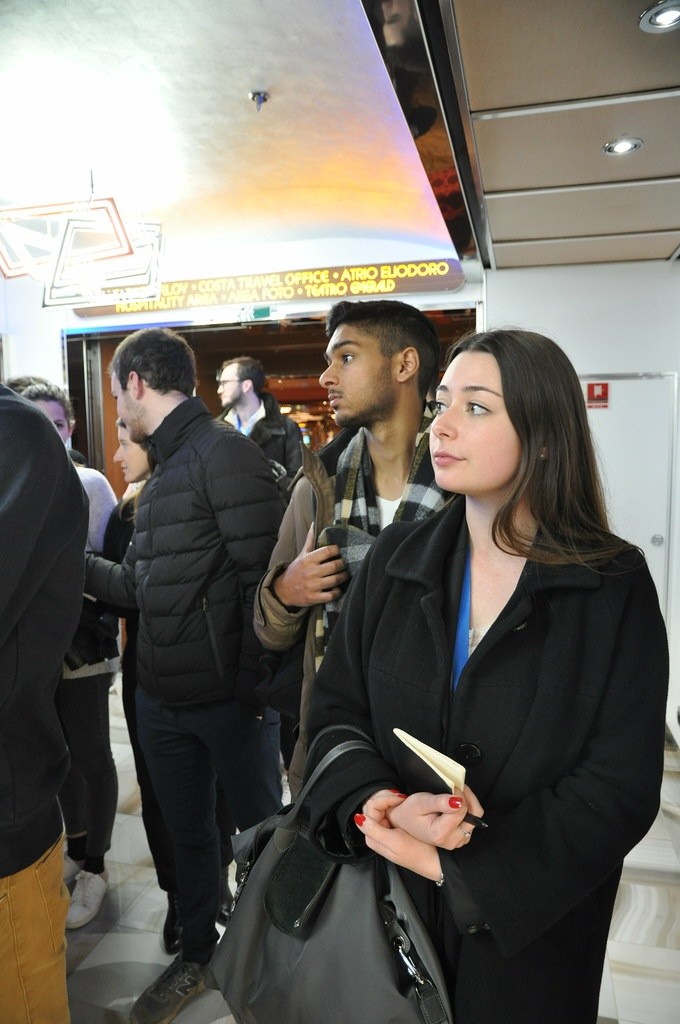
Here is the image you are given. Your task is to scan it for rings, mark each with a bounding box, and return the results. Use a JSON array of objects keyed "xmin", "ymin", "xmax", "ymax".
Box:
[{"xmin": 461, "ymin": 827, "xmax": 471, "ymax": 838}]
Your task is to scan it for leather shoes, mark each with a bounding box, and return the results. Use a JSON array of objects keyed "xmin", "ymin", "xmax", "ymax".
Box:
[
  {"xmin": 163, "ymin": 890, "xmax": 184, "ymax": 954},
  {"xmin": 216, "ymin": 865, "xmax": 233, "ymax": 926}
]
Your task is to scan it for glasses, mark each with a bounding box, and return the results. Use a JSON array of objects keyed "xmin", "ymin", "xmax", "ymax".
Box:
[{"xmin": 217, "ymin": 380, "xmax": 244, "ymax": 387}]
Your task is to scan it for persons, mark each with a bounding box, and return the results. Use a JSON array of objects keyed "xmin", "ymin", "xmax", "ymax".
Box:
[
  {"xmin": 0, "ymin": 327, "xmax": 304, "ymax": 1023},
  {"xmin": 305, "ymin": 324, "xmax": 670, "ymax": 1024},
  {"xmin": 251, "ymin": 299, "xmax": 458, "ymax": 801}
]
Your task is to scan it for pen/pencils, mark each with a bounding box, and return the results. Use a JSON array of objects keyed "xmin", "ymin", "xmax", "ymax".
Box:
[{"xmin": 462, "ymin": 812, "xmax": 490, "ymax": 830}]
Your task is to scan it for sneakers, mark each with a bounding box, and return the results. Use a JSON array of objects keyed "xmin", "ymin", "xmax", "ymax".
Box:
[
  {"xmin": 130, "ymin": 943, "xmax": 220, "ymax": 1024},
  {"xmin": 66, "ymin": 867, "xmax": 108, "ymax": 930},
  {"xmin": 61, "ymin": 851, "xmax": 85, "ymax": 885}
]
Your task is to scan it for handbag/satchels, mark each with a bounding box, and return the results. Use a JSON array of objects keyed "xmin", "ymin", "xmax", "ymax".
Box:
[
  {"xmin": 209, "ymin": 725, "xmax": 453, "ymax": 1024},
  {"xmin": 229, "ymin": 804, "xmax": 294, "ymax": 890}
]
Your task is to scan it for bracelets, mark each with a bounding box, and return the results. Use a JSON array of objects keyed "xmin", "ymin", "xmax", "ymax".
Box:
[{"xmin": 436, "ymin": 871, "xmax": 444, "ymax": 887}]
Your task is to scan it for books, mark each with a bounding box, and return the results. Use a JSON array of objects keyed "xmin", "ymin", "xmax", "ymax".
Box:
[{"xmin": 391, "ymin": 728, "xmax": 467, "ymax": 797}]
